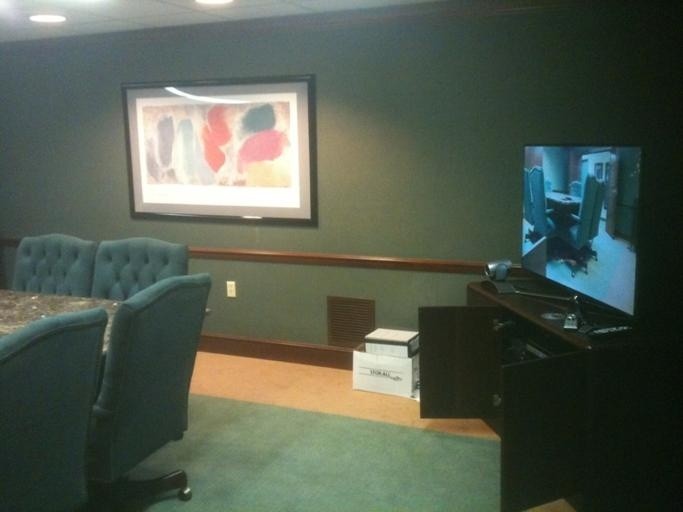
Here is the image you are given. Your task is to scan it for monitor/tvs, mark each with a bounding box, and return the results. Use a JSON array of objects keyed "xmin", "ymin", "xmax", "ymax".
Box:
[{"xmin": 520, "ymin": 143, "xmax": 646, "ymax": 336}]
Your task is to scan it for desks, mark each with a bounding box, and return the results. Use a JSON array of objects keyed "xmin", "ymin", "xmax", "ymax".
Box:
[{"xmin": 0, "ymin": 288, "xmax": 125, "ymax": 356}]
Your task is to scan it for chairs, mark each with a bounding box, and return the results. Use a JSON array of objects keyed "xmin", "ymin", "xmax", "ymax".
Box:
[
  {"xmin": 92, "ymin": 235, "xmax": 188, "ymax": 300},
  {"xmin": 11, "ymin": 233, "xmax": 97, "ymax": 297},
  {"xmin": 2, "ymin": 307, "xmax": 107, "ymax": 512},
  {"xmin": 523, "ymin": 163, "xmax": 606, "ymax": 278},
  {"xmin": 92, "ymin": 271, "xmax": 213, "ymax": 512}
]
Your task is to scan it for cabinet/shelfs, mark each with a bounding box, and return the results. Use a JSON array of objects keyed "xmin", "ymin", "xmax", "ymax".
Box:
[{"xmin": 418, "ymin": 277, "xmax": 629, "ymax": 512}]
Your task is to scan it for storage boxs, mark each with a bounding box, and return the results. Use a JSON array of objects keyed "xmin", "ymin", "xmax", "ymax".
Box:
[
  {"xmin": 364, "ymin": 328, "xmax": 419, "ymax": 358},
  {"xmin": 351, "ymin": 343, "xmax": 420, "ymax": 398}
]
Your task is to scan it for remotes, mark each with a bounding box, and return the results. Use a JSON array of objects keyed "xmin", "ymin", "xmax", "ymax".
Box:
[{"xmin": 563, "ymin": 312, "xmax": 579, "ymax": 331}]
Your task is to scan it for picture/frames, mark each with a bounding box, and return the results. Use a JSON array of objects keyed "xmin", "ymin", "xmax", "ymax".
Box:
[{"xmin": 120, "ymin": 75, "xmax": 318, "ymax": 227}]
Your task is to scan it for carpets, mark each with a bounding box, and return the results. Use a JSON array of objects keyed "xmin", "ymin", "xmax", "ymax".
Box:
[{"xmin": 132, "ymin": 392, "xmax": 502, "ymax": 512}]
